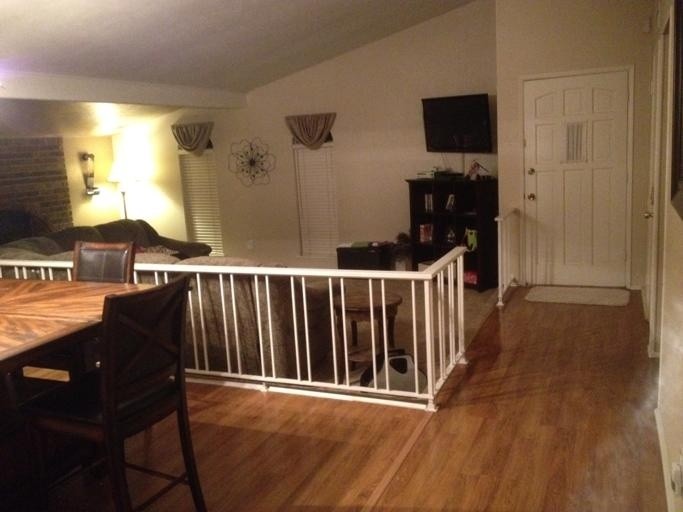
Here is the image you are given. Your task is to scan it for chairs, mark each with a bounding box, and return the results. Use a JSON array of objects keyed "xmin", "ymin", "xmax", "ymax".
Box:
[
  {"xmin": 11, "ymin": 274, "xmax": 206, "ymax": 511},
  {"xmin": 73, "ymin": 240, "xmax": 135, "ymax": 284}
]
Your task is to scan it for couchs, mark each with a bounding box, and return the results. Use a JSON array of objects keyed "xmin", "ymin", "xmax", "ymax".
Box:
[{"xmin": 0, "ymin": 218, "xmax": 345, "ymax": 382}]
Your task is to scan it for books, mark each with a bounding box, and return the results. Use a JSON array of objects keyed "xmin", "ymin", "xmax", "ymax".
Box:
[
  {"xmin": 425, "ymin": 194, "xmax": 433, "ymax": 212},
  {"xmin": 420, "ymin": 224, "xmax": 433, "ymax": 243},
  {"xmin": 446, "ymin": 194, "xmax": 455, "ymax": 211}
]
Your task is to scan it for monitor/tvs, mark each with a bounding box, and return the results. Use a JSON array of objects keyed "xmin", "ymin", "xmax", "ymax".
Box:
[{"xmin": 421, "ymin": 93, "xmax": 491, "ymax": 153}]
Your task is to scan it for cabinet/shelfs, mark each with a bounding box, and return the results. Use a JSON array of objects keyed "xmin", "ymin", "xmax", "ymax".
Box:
[{"xmin": 406, "ymin": 176, "xmax": 497, "ymax": 292}]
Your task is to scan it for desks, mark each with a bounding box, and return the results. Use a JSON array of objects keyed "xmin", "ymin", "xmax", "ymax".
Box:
[{"xmin": 1, "ymin": 277, "xmax": 194, "ymax": 417}]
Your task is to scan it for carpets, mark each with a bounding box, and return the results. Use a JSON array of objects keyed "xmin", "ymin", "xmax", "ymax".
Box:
[{"xmin": 524, "ymin": 286, "xmax": 631, "ymax": 307}]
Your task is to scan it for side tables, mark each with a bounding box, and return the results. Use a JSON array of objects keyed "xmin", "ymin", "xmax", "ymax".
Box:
[{"xmin": 334, "ymin": 290, "xmax": 402, "ymax": 382}]
